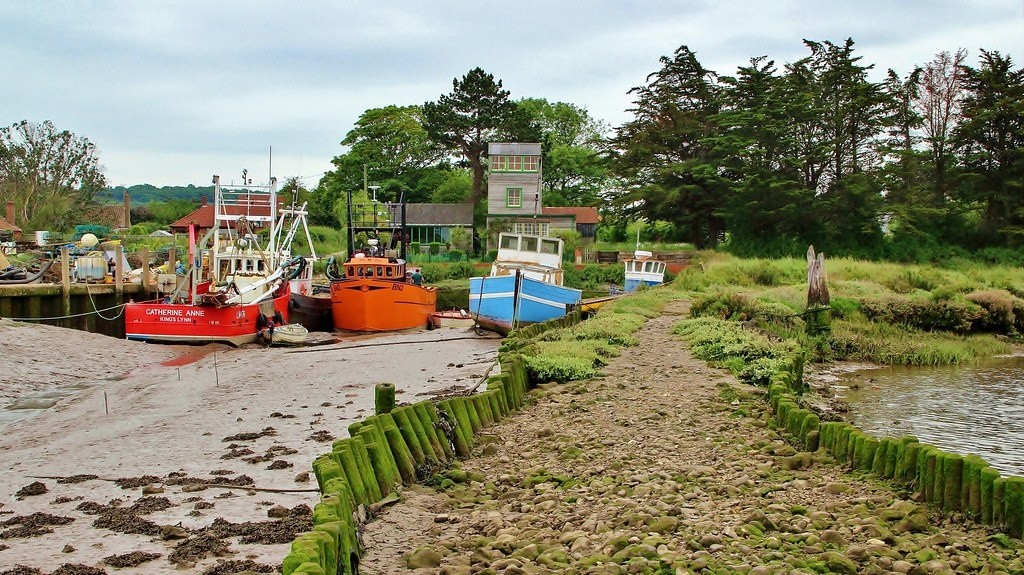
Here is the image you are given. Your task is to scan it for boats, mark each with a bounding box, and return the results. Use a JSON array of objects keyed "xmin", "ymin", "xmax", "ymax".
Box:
[
  {"xmin": 621, "ymin": 251, "xmax": 667, "ymax": 294},
  {"xmin": 468, "ymin": 232, "xmax": 583, "ymax": 338},
  {"xmin": 287, "ymin": 289, "xmax": 333, "ymax": 331},
  {"xmin": 324, "ymin": 184, "xmax": 437, "ymax": 334},
  {"xmin": 256, "ymin": 323, "xmax": 309, "ymax": 346},
  {"xmin": 429, "ymin": 310, "xmax": 477, "ymax": 331},
  {"xmin": 278, "ymin": 201, "xmax": 319, "ymax": 296},
  {"xmin": 123, "ymin": 145, "xmax": 294, "ymax": 348}
]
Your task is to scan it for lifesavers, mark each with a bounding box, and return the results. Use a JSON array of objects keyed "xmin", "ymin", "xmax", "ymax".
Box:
[{"xmin": 300, "ymin": 282, "xmax": 307, "ymax": 296}]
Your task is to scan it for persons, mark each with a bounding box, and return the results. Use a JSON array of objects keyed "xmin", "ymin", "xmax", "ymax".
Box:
[
  {"xmin": 108, "ymin": 257, "xmax": 116, "ymax": 278},
  {"xmin": 409, "ymin": 269, "xmax": 424, "ymax": 286}
]
